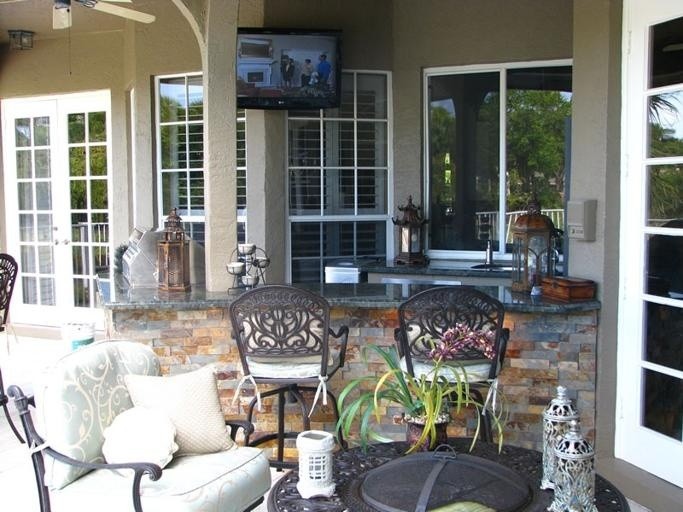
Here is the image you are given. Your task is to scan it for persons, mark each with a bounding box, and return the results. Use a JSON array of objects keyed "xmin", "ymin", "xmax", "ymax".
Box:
[{"xmin": 280, "ymin": 53, "xmax": 330, "ymax": 92}]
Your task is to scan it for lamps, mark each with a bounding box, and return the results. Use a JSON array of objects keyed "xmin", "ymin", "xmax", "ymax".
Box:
[
  {"xmin": 390, "ymin": 194, "xmax": 431, "ymax": 266},
  {"xmin": 510, "ymin": 201, "xmax": 557, "ymax": 294}
]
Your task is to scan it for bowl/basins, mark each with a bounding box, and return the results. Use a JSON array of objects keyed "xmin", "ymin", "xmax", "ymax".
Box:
[{"xmin": 227, "ymin": 244, "xmax": 270, "ymax": 287}]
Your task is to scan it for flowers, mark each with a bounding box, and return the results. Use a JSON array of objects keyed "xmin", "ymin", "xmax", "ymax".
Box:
[{"xmin": 337, "ymin": 322, "xmax": 509, "ymax": 455}]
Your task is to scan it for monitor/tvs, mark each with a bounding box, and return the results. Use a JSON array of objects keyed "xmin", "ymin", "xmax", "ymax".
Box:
[{"xmin": 236, "ymin": 26, "xmax": 343, "ymax": 111}]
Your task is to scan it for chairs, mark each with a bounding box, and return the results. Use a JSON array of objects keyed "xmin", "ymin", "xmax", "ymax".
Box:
[
  {"xmin": 392, "ymin": 286, "xmax": 511, "ymax": 444},
  {"xmin": 7, "ymin": 341, "xmax": 270, "ymax": 510},
  {"xmin": 230, "ymin": 287, "xmax": 350, "ymax": 475}
]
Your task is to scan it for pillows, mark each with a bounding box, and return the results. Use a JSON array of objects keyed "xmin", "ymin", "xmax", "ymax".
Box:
[{"xmin": 96, "ymin": 368, "xmax": 235, "ymax": 476}]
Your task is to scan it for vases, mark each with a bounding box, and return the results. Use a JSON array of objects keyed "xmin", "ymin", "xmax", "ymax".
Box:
[{"xmin": 405, "ymin": 421, "xmax": 450, "ymax": 451}]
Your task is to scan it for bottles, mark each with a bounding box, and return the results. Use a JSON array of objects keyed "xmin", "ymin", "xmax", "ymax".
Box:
[{"xmin": 486, "ymin": 241, "xmax": 494, "ymax": 265}]
